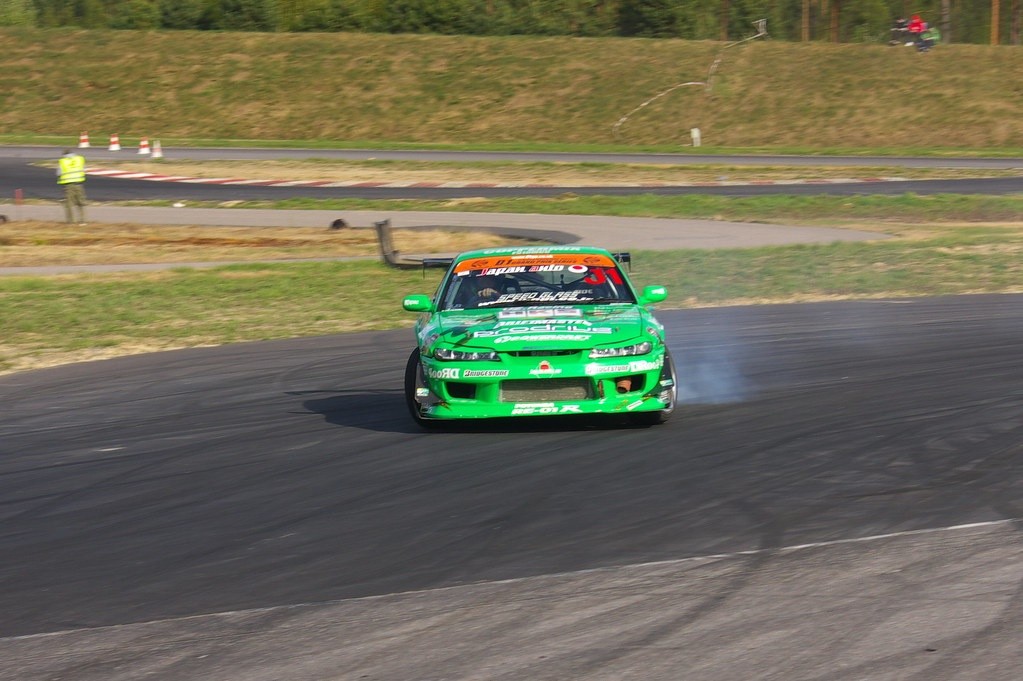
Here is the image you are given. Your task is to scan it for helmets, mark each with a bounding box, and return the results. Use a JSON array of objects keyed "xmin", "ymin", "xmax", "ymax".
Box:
[{"xmin": 471, "ymin": 270, "xmax": 506, "ymax": 298}]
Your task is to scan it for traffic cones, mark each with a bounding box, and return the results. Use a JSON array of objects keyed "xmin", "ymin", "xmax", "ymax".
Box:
[
  {"xmin": 76, "ymin": 130, "xmax": 93, "ymax": 150},
  {"xmin": 137, "ymin": 137, "xmax": 151, "ymax": 156},
  {"xmin": 150, "ymin": 139, "xmax": 166, "ymax": 159},
  {"xmin": 108, "ymin": 132, "xmax": 122, "ymax": 152}
]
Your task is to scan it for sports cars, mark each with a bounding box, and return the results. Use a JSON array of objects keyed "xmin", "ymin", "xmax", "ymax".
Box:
[{"xmin": 403, "ymin": 246, "xmax": 680, "ymax": 428}]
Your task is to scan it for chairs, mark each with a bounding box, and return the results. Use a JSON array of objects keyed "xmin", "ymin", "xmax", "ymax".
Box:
[{"xmin": 574, "ymin": 282, "xmax": 609, "ymax": 299}]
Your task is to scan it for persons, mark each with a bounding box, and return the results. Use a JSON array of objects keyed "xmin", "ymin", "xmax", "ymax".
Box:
[
  {"xmin": 889, "ymin": 13, "xmax": 941, "ymax": 51},
  {"xmin": 454, "ymin": 270, "xmax": 502, "ymax": 308},
  {"xmin": 57, "ymin": 149, "xmax": 87, "ymax": 225}
]
